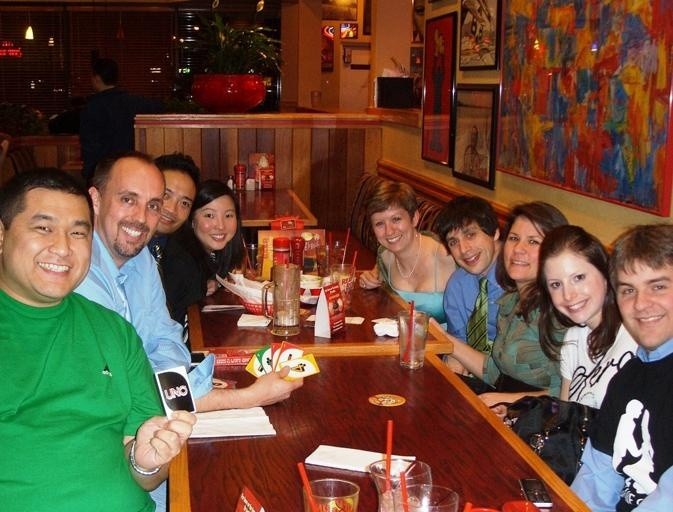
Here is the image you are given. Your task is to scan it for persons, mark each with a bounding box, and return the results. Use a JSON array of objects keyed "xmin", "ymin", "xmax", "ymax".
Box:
[
  {"xmin": 146, "ymin": 150, "xmax": 201, "ymax": 327},
  {"xmin": 428, "ymin": 33, "xmax": 445, "ymax": 155},
  {"xmin": 426, "ymin": 199, "xmax": 570, "ymax": 410},
  {"xmin": 487, "ymin": 221, "xmax": 639, "ymax": 490},
  {"xmin": 70, "ymin": 150, "xmax": 305, "ymax": 415},
  {"xmin": 461, "ymin": 126, "xmax": 482, "ymax": 176},
  {"xmin": 356, "ymin": 179, "xmax": 461, "ymax": 337},
  {"xmin": 168, "ymin": 179, "xmax": 250, "ymax": 298},
  {"xmin": 569, "ymin": 221, "xmax": 671, "ymax": 511},
  {"xmin": 49, "ymin": 58, "xmax": 181, "ymax": 182},
  {"xmin": 0, "ymin": 167, "xmax": 196, "ymax": 512},
  {"xmin": 436, "ymin": 192, "xmax": 505, "ymax": 379}
]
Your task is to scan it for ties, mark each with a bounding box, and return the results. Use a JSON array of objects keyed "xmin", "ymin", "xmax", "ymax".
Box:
[
  {"xmin": 466, "ymin": 278, "xmax": 490, "ymax": 379},
  {"xmin": 155, "ymin": 241, "xmax": 164, "ymax": 281}
]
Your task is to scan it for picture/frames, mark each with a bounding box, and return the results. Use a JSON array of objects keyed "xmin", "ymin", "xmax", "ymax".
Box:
[
  {"xmin": 421, "ymin": 11, "xmax": 458, "ymax": 167},
  {"xmin": 495, "ymin": 0, "xmax": 673, "ymax": 217},
  {"xmin": 451, "ymin": 82, "xmax": 500, "ymax": 189},
  {"xmin": 458, "ymin": 0, "xmax": 499, "ymax": 70}
]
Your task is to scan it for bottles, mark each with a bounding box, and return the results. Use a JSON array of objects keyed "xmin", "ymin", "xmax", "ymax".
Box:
[{"xmin": 224, "ymin": 160, "xmax": 257, "ymax": 191}]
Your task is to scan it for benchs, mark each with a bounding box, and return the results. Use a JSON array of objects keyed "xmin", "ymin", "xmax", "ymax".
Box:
[{"xmin": 325, "ymin": 158, "xmax": 615, "ymax": 300}]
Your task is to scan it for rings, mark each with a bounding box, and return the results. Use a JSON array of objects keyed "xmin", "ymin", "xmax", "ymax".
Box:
[{"xmin": 363, "ymin": 282, "xmax": 366, "ymax": 288}]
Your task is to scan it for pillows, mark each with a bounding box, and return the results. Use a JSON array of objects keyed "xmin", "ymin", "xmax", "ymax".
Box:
[{"xmin": 348, "ymin": 173, "xmax": 440, "ymax": 251}]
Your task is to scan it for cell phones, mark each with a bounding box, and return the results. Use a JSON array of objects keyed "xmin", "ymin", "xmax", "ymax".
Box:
[{"xmin": 518, "ymin": 476, "xmax": 554, "ymax": 508}]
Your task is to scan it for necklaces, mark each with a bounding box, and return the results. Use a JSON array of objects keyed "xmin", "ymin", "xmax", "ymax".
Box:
[{"xmin": 394, "ymin": 232, "xmax": 422, "ymax": 281}]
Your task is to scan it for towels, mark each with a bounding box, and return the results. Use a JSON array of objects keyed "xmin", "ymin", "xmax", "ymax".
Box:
[
  {"xmin": 236, "ymin": 308, "xmax": 272, "ymax": 327},
  {"xmin": 372, "ymin": 316, "xmax": 449, "ymax": 338},
  {"xmin": 188, "ymin": 405, "xmax": 277, "ymax": 440},
  {"xmin": 306, "ymin": 313, "xmax": 366, "ymax": 325},
  {"xmin": 304, "ymin": 443, "xmax": 416, "ymax": 481}
]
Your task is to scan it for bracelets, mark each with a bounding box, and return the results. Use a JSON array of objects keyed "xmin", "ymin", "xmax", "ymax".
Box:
[{"xmin": 127, "ymin": 437, "xmax": 162, "ymax": 478}]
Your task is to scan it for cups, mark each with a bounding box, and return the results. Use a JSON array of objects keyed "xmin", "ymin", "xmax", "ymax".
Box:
[
  {"xmin": 301, "ymin": 457, "xmax": 460, "ymax": 512},
  {"xmin": 396, "ymin": 310, "xmax": 429, "ymax": 370},
  {"xmin": 261, "ymin": 214, "xmax": 358, "ymax": 339},
  {"xmin": 309, "ymin": 90, "xmax": 324, "ymax": 107}
]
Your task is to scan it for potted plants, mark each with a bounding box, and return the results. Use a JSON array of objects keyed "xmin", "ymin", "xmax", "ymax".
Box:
[{"xmin": 191, "ymin": 11, "xmax": 286, "ymax": 114}]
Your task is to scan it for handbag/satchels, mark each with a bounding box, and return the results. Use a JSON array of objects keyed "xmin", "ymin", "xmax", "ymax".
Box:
[{"xmin": 502, "ymin": 395, "xmax": 601, "ymax": 487}]
